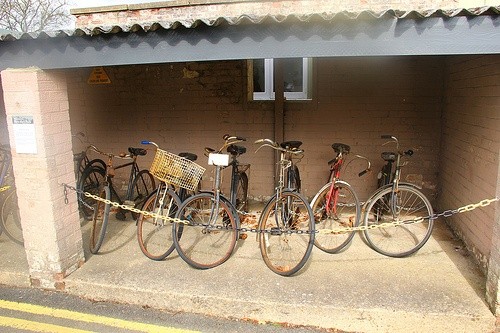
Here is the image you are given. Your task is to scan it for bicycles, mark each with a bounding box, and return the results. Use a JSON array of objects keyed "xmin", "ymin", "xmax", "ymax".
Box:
[{"xmin": 0, "ymin": 130, "xmax": 434, "ymax": 278}]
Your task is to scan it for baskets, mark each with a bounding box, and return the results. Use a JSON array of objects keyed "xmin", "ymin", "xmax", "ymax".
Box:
[{"xmin": 149, "ymin": 147, "xmax": 206, "ymax": 192}]
